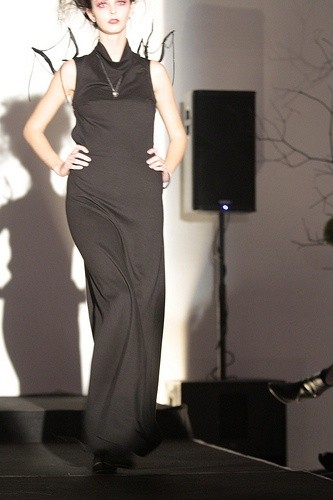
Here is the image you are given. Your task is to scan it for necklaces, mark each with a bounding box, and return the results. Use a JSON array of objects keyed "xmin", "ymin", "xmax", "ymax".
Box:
[{"xmin": 94, "ymin": 48, "xmax": 130, "ymax": 97}]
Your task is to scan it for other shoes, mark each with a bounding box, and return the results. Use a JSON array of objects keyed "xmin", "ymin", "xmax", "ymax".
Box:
[{"xmin": 92, "ymin": 462, "xmax": 116, "ymax": 473}]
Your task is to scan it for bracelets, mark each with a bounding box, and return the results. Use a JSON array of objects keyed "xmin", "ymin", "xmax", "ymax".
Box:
[{"xmin": 163, "ymin": 171, "xmax": 171, "ymax": 189}]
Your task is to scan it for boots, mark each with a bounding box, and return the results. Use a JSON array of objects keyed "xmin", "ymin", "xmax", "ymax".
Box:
[{"xmin": 267, "ymin": 369, "xmax": 332, "ymax": 405}]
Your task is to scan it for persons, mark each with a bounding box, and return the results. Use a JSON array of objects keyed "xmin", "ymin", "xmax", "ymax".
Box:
[{"xmin": 23, "ymin": 0, "xmax": 188, "ymax": 474}]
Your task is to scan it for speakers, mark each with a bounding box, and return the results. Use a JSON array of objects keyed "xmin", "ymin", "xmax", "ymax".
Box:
[
  {"xmin": 182, "ymin": 379, "xmax": 287, "ymax": 467},
  {"xmin": 192, "ymin": 90, "xmax": 256, "ymax": 212}
]
